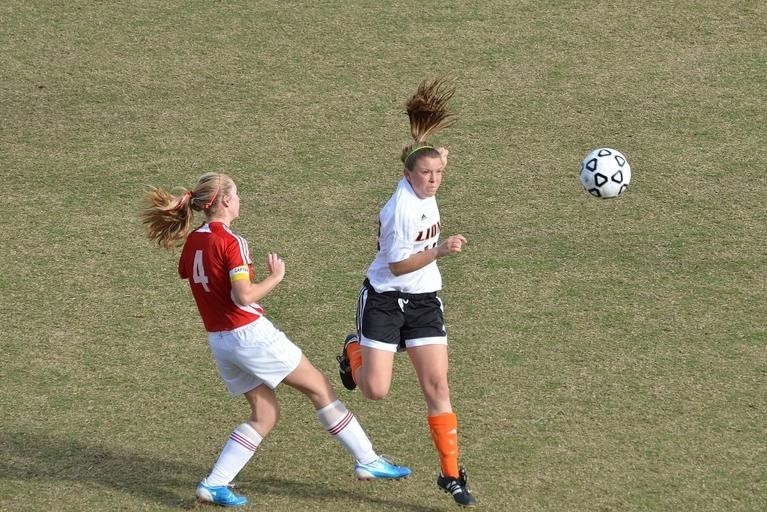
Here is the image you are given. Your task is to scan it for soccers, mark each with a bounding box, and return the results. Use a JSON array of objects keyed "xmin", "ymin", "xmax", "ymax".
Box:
[{"xmin": 579, "ymin": 147, "xmax": 631, "ymax": 198}]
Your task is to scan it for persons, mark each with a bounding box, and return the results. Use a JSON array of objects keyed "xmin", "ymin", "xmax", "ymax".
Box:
[
  {"xmin": 140, "ymin": 172, "xmax": 411, "ymax": 507},
  {"xmin": 340, "ymin": 80, "xmax": 478, "ymax": 507}
]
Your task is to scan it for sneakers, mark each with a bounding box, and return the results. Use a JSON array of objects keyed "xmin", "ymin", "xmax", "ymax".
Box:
[
  {"xmin": 353, "ymin": 456, "xmax": 412, "ymax": 481},
  {"xmin": 336, "ymin": 334, "xmax": 357, "ymax": 391},
  {"xmin": 196, "ymin": 478, "xmax": 248, "ymax": 507},
  {"xmin": 438, "ymin": 468, "xmax": 476, "ymax": 507}
]
